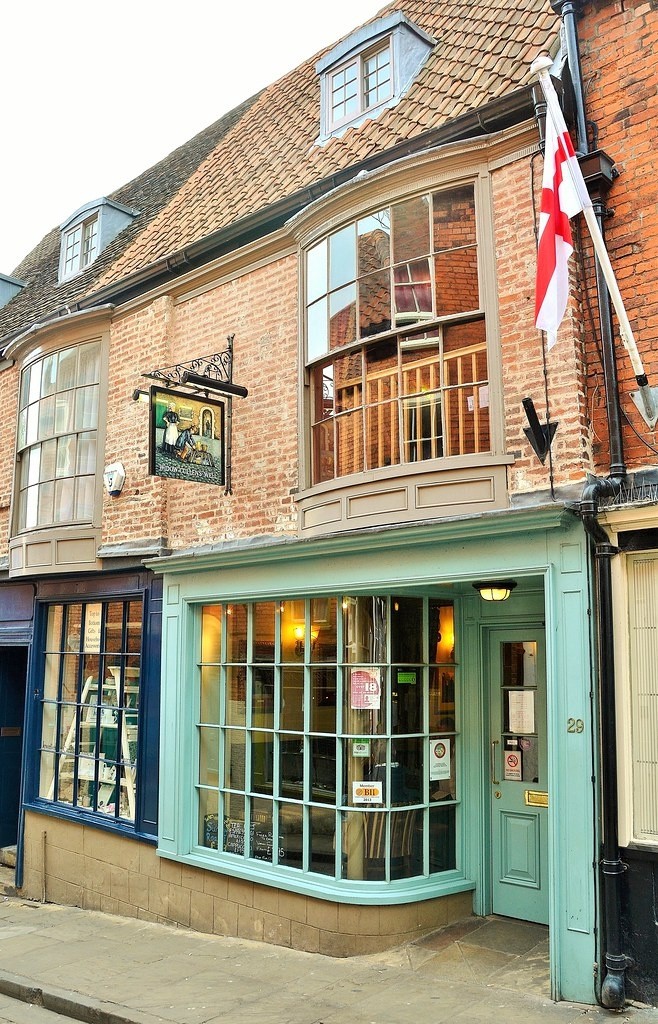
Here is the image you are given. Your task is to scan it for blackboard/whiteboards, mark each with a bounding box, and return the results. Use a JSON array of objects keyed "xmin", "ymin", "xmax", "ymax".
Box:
[{"xmin": 204, "ymin": 813, "xmax": 284, "ymax": 864}]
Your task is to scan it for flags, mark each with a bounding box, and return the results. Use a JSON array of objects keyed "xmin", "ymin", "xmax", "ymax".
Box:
[{"xmin": 535, "ymin": 82, "xmax": 592, "ymax": 348}]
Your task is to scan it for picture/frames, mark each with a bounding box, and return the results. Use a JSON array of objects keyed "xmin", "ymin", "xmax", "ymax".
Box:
[{"xmin": 434, "ymin": 666, "xmax": 455, "ymax": 715}]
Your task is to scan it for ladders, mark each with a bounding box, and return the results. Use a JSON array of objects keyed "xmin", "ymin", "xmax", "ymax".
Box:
[{"xmin": 44, "ymin": 670, "xmax": 140, "ymax": 821}]
